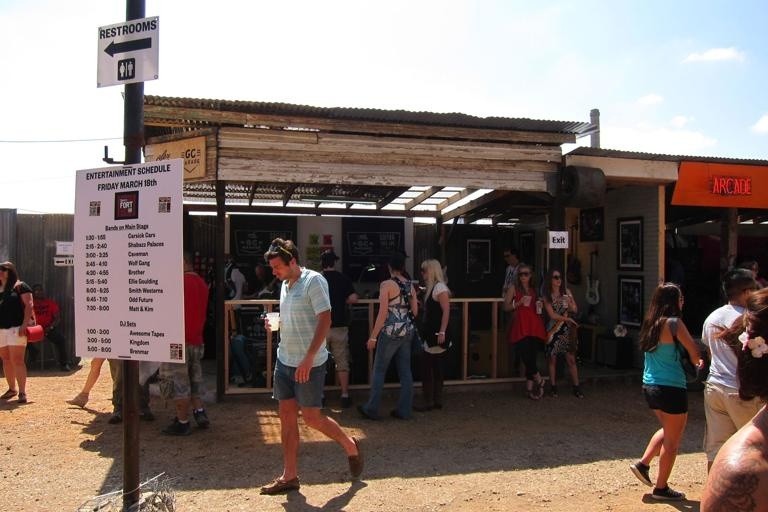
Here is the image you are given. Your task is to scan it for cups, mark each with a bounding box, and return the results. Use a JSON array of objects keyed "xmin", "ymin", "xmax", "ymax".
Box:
[
  {"xmin": 266, "ymin": 311, "xmax": 279, "ymax": 332},
  {"xmin": 535, "ymin": 300, "xmax": 542, "ymax": 315},
  {"xmin": 562, "ymin": 295, "xmax": 569, "ymax": 309}
]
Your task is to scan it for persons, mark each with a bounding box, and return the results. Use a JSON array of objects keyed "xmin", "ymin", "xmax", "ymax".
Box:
[
  {"xmin": 697, "ymin": 285, "xmax": 768, "ymax": 511},
  {"xmin": 161, "ymin": 255, "xmax": 210, "ymax": 435},
  {"xmin": 702, "ymin": 267, "xmax": 757, "ymax": 480},
  {"xmin": 224, "ymin": 255, "xmax": 282, "ymax": 387},
  {"xmin": 503, "ymin": 248, "xmax": 584, "ymax": 400},
  {"xmin": 127, "ymin": 61, "xmax": 134, "ymax": 77},
  {"xmin": 413, "ymin": 258, "xmax": 454, "ymax": 411},
  {"xmin": 118, "ymin": 61, "xmax": 126, "ymax": 79},
  {"xmin": 261, "ymin": 237, "xmax": 362, "ymax": 496},
  {"xmin": 321, "ymin": 250, "xmax": 357, "ymax": 409},
  {"xmin": 629, "ymin": 281, "xmax": 707, "ymax": 499},
  {"xmin": 739, "ymin": 261, "xmax": 768, "ymax": 286},
  {"xmin": 28, "ymin": 284, "xmax": 73, "ymax": 371},
  {"xmin": 65, "ymin": 357, "xmax": 106, "ymax": 409},
  {"xmin": 358, "ymin": 252, "xmax": 418, "ymax": 418},
  {"xmin": 0, "ymin": 259, "xmax": 35, "ymax": 403},
  {"xmin": 109, "ymin": 355, "xmax": 153, "ymax": 424}
]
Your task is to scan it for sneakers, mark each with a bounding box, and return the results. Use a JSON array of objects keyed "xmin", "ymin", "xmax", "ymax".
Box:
[
  {"xmin": 107, "ymin": 416, "xmax": 120, "ymax": 424},
  {"xmin": 630, "ymin": 462, "xmax": 652, "ymax": 487},
  {"xmin": 0, "ymin": 389, "xmax": 17, "ymax": 399},
  {"xmin": 652, "ymin": 485, "xmax": 685, "ymax": 500},
  {"xmin": 17, "ymin": 392, "xmax": 27, "ymax": 403},
  {"xmin": 140, "ymin": 412, "xmax": 156, "ymax": 422},
  {"xmin": 340, "ymin": 395, "xmax": 352, "ymax": 407}
]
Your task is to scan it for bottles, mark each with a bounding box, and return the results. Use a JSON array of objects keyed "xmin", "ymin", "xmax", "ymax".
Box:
[{"xmin": 524, "ymin": 288, "xmax": 530, "ymax": 308}]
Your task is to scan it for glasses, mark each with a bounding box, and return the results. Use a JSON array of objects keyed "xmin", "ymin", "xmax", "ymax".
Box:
[
  {"xmin": 518, "ymin": 272, "xmax": 529, "ymax": 277},
  {"xmin": 553, "ymin": 275, "xmax": 561, "ymax": 280},
  {"xmin": 269, "ymin": 244, "xmax": 293, "ymax": 259}
]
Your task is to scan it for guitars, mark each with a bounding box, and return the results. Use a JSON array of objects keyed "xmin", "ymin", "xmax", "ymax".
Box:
[
  {"xmin": 566, "ymin": 227, "xmax": 580, "ymax": 284},
  {"xmin": 586, "ymin": 252, "xmax": 600, "ymax": 306}
]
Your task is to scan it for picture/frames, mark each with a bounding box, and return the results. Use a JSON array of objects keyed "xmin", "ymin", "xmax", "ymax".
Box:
[
  {"xmin": 580, "ymin": 206, "xmax": 604, "ymax": 241},
  {"xmin": 617, "ymin": 216, "xmax": 643, "ymax": 269},
  {"xmin": 464, "ymin": 237, "xmax": 494, "ymax": 275},
  {"xmin": 617, "ymin": 274, "xmax": 644, "ymax": 329}
]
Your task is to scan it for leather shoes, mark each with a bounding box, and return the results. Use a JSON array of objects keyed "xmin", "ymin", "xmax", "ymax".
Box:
[
  {"xmin": 193, "ymin": 407, "xmax": 209, "ymax": 426},
  {"xmin": 357, "ymin": 404, "xmax": 376, "ymax": 420},
  {"xmin": 261, "ymin": 477, "xmax": 300, "ymax": 494},
  {"xmin": 161, "ymin": 420, "xmax": 192, "ymax": 434},
  {"xmin": 389, "ymin": 406, "xmax": 407, "ymax": 419},
  {"xmin": 348, "ymin": 436, "xmax": 364, "ymax": 477}
]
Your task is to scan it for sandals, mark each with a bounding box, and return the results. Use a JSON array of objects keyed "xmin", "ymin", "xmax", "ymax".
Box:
[
  {"xmin": 66, "ymin": 393, "xmax": 89, "ymax": 408},
  {"xmin": 573, "ymin": 391, "xmax": 585, "ymax": 399},
  {"xmin": 526, "ymin": 379, "xmax": 559, "ymax": 401}
]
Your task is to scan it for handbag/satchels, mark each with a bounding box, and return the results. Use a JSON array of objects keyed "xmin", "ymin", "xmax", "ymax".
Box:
[
  {"xmin": 412, "ymin": 328, "xmax": 425, "ymax": 355},
  {"xmin": 26, "ymin": 324, "xmax": 44, "ymax": 343},
  {"xmin": 668, "ymin": 317, "xmax": 710, "ymax": 386}
]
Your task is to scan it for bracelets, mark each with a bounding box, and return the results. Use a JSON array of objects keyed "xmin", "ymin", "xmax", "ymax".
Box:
[
  {"xmin": 439, "ymin": 332, "xmax": 445, "ymax": 335},
  {"xmin": 370, "ymin": 338, "xmax": 377, "ymax": 342},
  {"xmin": 49, "ymin": 325, "xmax": 55, "ymax": 330}
]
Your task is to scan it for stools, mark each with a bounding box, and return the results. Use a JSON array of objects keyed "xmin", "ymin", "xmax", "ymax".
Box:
[{"xmin": 26, "ymin": 338, "xmax": 56, "ymax": 371}]
